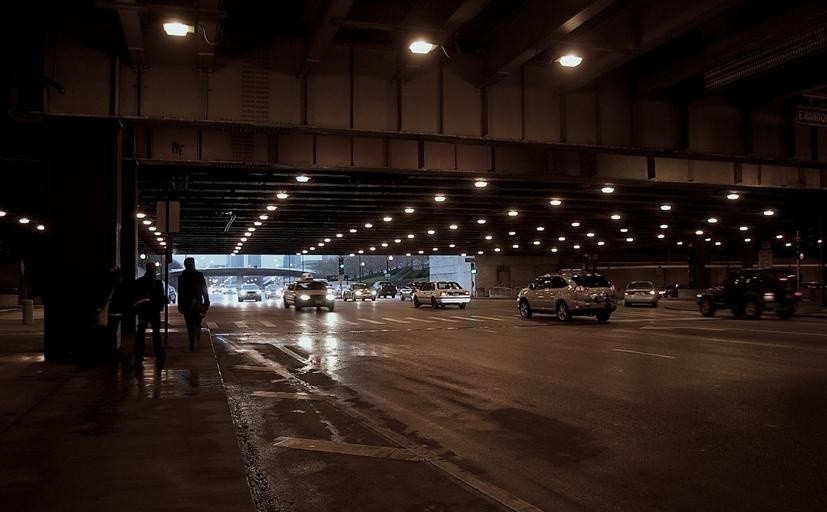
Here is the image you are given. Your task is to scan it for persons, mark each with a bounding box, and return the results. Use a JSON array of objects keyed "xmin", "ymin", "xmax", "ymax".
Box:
[
  {"xmin": 134, "ymin": 261, "xmax": 167, "ymax": 361},
  {"xmin": 177, "ymin": 257, "xmax": 210, "ymax": 352}
]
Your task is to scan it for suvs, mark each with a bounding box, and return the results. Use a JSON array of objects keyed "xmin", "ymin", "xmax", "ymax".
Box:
[
  {"xmin": 695, "ymin": 267, "xmax": 803, "ymax": 320},
  {"xmin": 515, "ymin": 268, "xmax": 617, "ymax": 322}
]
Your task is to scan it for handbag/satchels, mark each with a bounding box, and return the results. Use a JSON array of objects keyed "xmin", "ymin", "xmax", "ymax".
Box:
[{"xmin": 98, "ymin": 301, "xmax": 111, "ymax": 326}]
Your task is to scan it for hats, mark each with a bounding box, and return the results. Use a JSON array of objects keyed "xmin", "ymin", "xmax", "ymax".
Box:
[{"xmin": 146, "ymin": 262, "xmax": 157, "ymax": 271}]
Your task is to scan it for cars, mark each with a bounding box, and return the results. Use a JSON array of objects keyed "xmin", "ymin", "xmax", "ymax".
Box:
[
  {"xmin": 805, "ymin": 281, "xmax": 825, "ymax": 291},
  {"xmin": 623, "ymin": 281, "xmax": 682, "ymax": 307},
  {"xmin": 208, "ymin": 271, "xmax": 472, "ymax": 312}
]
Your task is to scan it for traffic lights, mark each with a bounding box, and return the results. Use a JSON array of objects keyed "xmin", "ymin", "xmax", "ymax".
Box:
[{"xmin": 338, "ymin": 257, "xmax": 344, "ymax": 275}]
